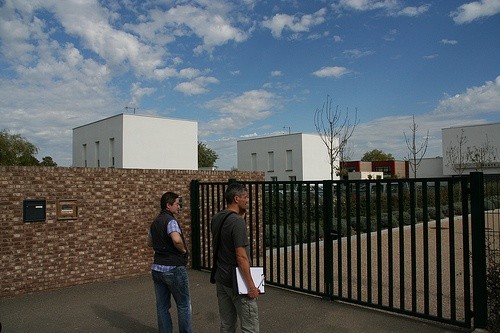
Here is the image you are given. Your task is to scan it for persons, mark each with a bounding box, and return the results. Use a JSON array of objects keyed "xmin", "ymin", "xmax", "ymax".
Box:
[
  {"xmin": 211, "ymin": 185, "xmax": 260, "ymax": 333},
  {"xmin": 148, "ymin": 192, "xmax": 192, "ymax": 333}
]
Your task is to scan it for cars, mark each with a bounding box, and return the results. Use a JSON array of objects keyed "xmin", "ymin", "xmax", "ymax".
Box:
[{"xmin": 265, "ymin": 182, "xmax": 421, "ymax": 201}]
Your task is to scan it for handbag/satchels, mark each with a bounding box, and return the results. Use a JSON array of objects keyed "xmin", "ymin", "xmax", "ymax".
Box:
[{"xmin": 210, "ymin": 267, "xmax": 217, "ymax": 284}]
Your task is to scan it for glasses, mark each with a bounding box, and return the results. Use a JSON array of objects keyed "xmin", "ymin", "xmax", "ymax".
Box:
[{"xmin": 172, "ymin": 203, "xmax": 180, "ymax": 206}]
